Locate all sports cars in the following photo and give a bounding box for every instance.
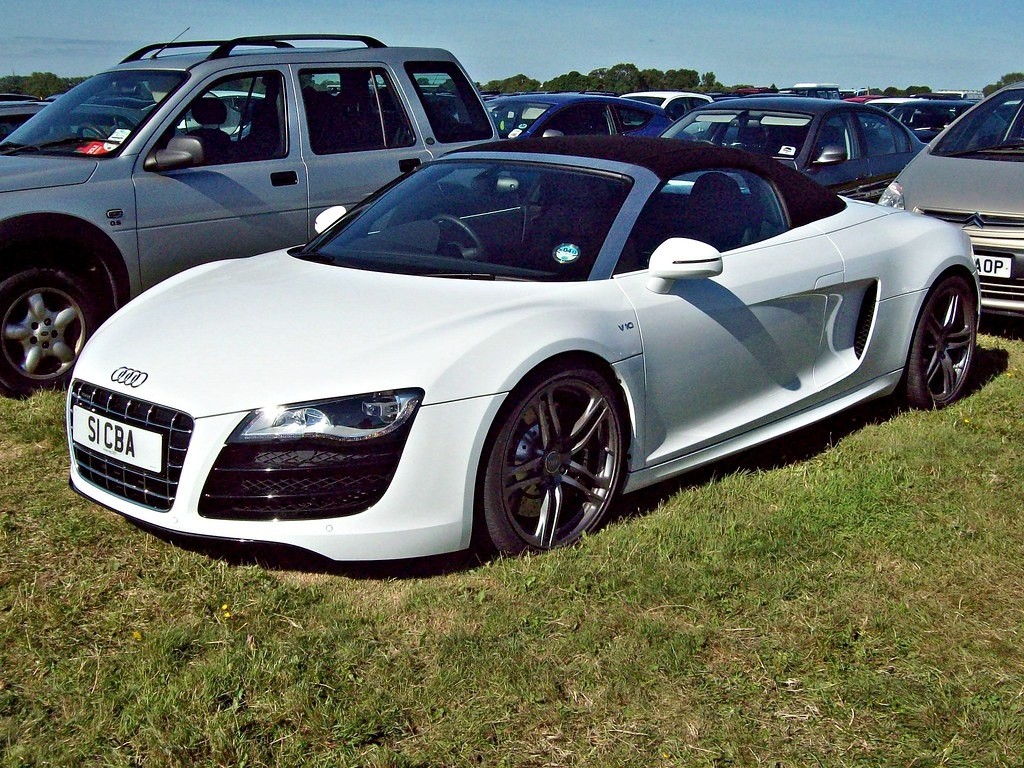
[65,134,981,564]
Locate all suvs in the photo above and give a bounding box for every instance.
[0,33,509,402]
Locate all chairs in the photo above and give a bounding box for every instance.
[672,104,685,120]
[736,127,768,153]
[185,98,234,159]
[794,126,823,160]
[225,98,280,161]
[684,171,748,251]
[480,190,535,256]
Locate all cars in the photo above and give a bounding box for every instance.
[0,74,1023,150]
[641,95,929,204]
[876,79,1023,321]
